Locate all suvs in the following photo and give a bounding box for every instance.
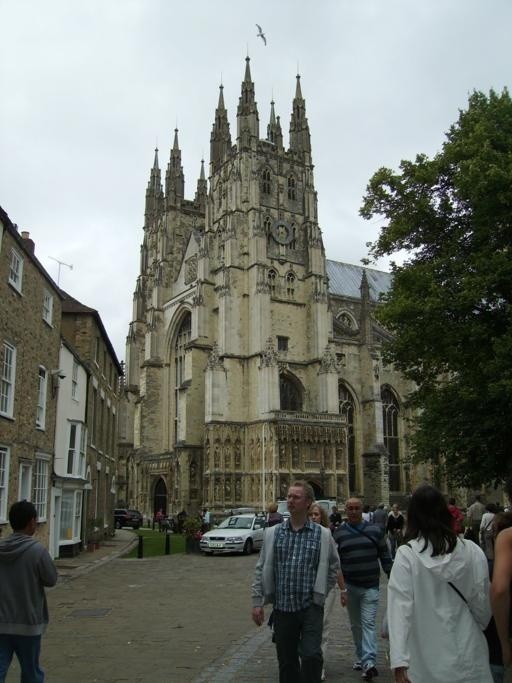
[114,509,143,530]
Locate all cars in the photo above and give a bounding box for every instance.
[199,512,268,556]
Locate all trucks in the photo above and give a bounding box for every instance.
[276,500,373,524]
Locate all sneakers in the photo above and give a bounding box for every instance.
[352,658,379,681]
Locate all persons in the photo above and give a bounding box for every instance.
[200,507,211,529]
[155,509,163,520]
[0,499,57,683]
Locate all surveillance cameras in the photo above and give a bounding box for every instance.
[57,371,66,379]
